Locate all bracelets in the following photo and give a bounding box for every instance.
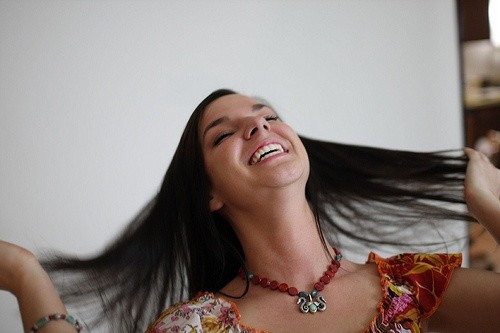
[25,312,83,333]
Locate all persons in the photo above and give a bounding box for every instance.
[1,88,499,332]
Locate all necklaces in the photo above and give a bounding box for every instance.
[247,247,343,313]
